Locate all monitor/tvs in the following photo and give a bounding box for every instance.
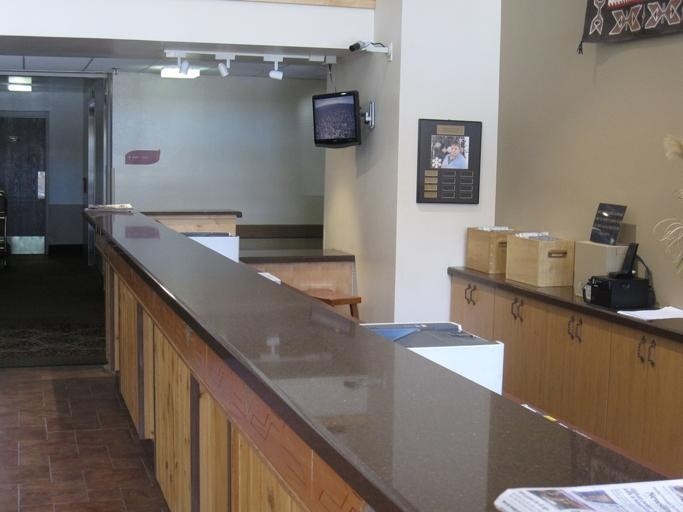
[312,91,375,148]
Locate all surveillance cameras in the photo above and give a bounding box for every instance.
[349,40,371,53]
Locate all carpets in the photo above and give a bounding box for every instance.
[0,243,108,370]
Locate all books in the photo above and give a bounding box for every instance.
[617,305,683,322]
[368,325,417,344]
[491,478,683,512]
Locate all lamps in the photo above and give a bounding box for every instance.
[175,51,285,80]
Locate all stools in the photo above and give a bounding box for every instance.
[304,287,361,320]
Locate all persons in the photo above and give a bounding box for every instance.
[438,140,467,171]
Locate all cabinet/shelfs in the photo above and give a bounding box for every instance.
[450,274,683,481]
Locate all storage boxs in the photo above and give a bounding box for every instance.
[465,227,574,288]
